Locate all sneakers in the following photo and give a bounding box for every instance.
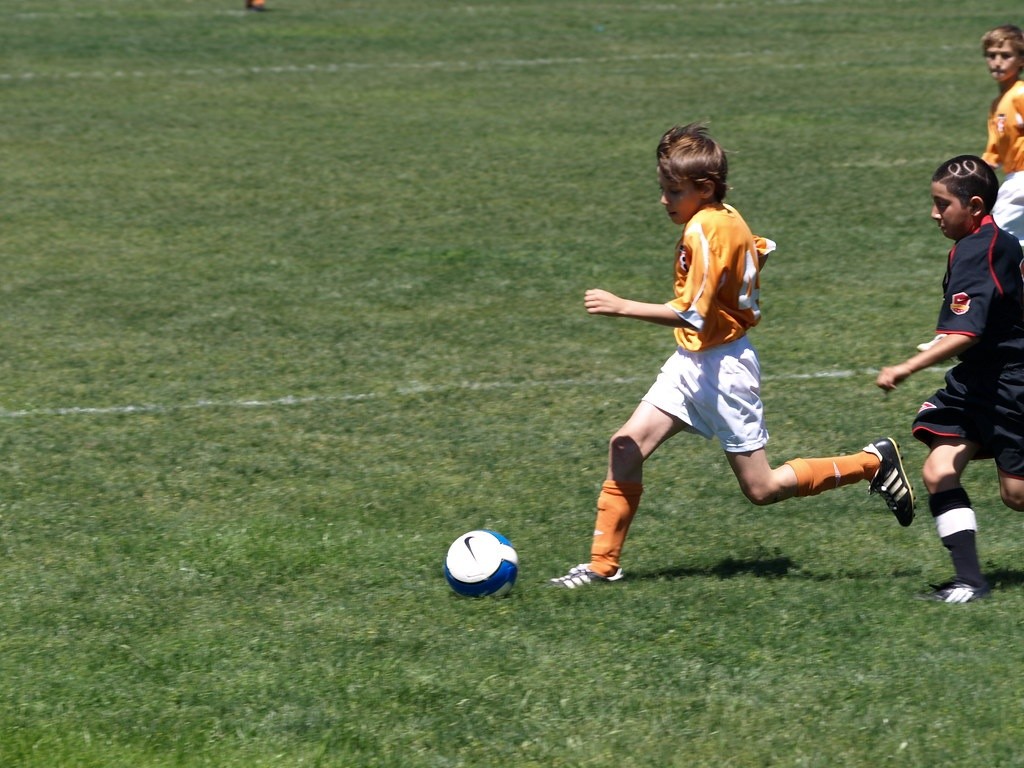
[914,576,987,603]
[550,563,623,588]
[863,437,916,527]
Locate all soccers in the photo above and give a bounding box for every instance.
[445,530,518,598]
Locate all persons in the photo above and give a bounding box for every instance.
[876,155,1024,602]
[551,124,916,591]
[914,25,1024,352]
